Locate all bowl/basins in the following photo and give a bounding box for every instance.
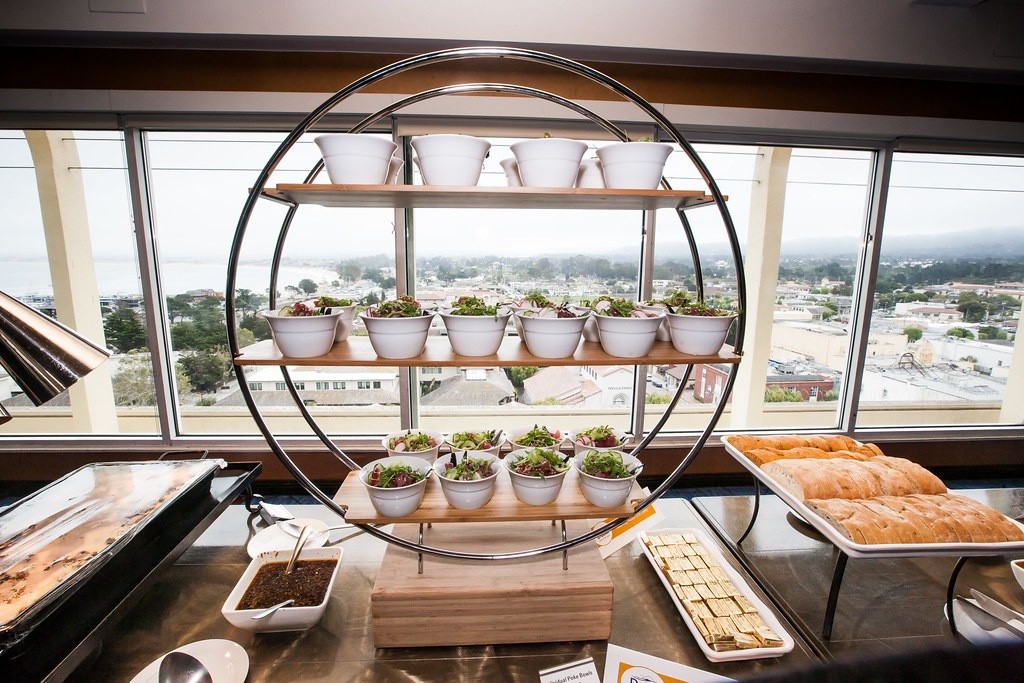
[1011,559,1024,591]
[313,133,674,191]
[358,428,644,518]
[261,298,741,359]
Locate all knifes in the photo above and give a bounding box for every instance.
[956,595,1024,640]
[970,588,1024,627]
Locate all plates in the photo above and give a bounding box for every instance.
[944,598,1024,647]
[720,433,1024,560]
[220,546,344,634]
[247,519,330,559]
[128,639,249,683]
[635,528,795,662]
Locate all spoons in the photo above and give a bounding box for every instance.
[158,652,213,683]
[276,520,356,539]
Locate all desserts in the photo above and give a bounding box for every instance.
[639,531,783,651]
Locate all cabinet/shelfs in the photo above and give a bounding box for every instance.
[226,45,746,656]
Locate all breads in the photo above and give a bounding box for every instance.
[727,433,1024,545]
